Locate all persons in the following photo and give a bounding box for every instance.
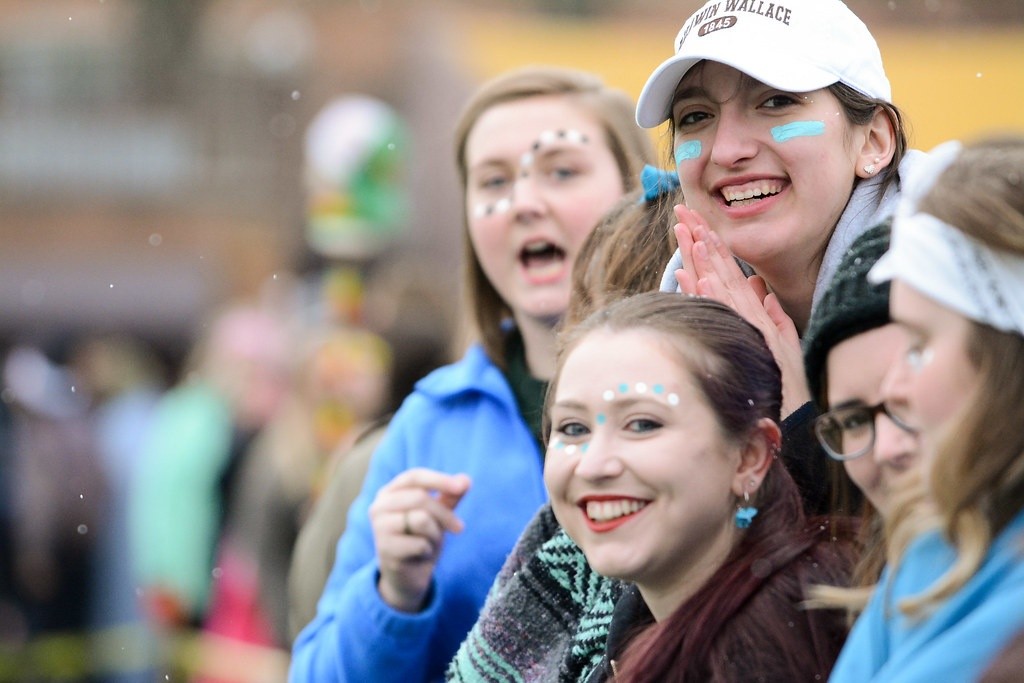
[543,292,867,682]
[451,163,760,683]
[635,1,912,519]
[0,91,452,683]
[288,70,659,682]
[804,215,926,622]
[827,146,1024,683]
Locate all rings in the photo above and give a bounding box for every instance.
[404,510,412,535]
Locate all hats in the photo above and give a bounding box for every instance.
[635,0,893,128]
[803,222,891,413]
[301,95,413,256]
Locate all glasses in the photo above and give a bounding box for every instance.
[810,399,917,462]
